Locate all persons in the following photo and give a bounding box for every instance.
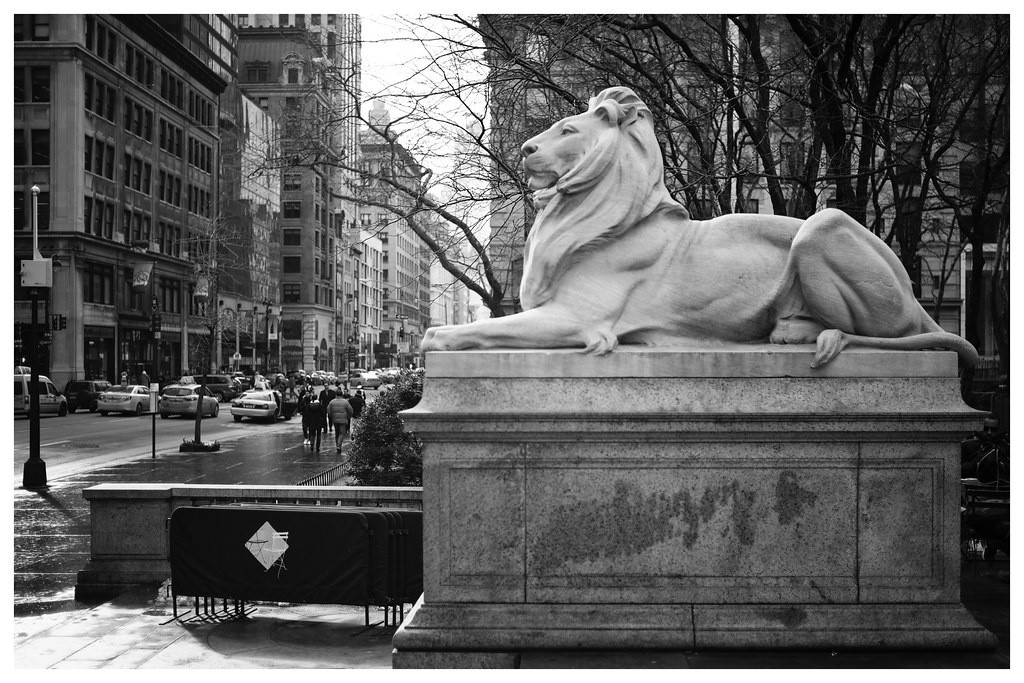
[961,415,1010,557]
[140,371,386,453]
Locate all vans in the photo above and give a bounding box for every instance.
[15,365,69,417]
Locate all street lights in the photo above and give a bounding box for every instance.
[18,184,53,488]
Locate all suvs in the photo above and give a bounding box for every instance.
[63,379,112,413]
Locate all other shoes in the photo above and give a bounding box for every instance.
[316,448,320,451]
[303,439,310,444]
[337,448,341,450]
[311,443,314,452]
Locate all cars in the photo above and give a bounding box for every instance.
[230,389,301,423]
[344,373,381,389]
[185,363,400,402]
[97,384,161,416]
[161,376,219,418]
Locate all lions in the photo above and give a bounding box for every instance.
[421,83,983,378]
